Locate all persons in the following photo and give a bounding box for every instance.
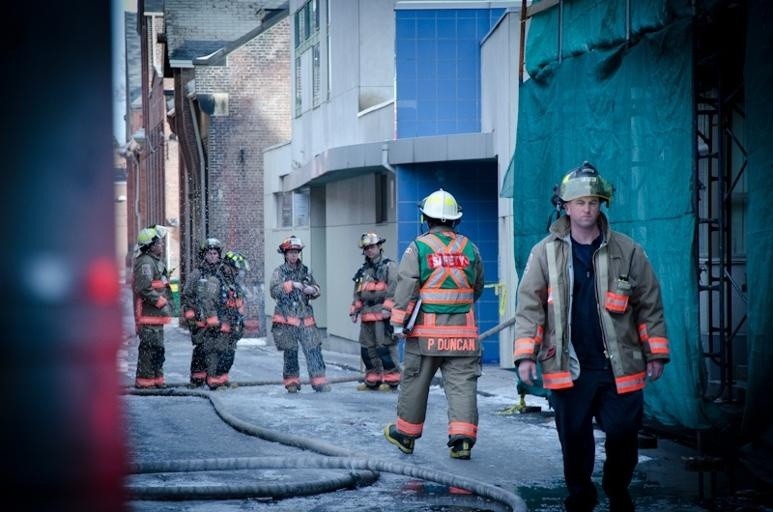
[182,237,223,386]
[205,250,251,389]
[269,235,333,392]
[385,187,485,460]
[513,161,673,511]
[131,224,176,389]
[349,233,401,391]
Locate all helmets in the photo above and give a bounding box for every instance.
[224,250,251,272]
[200,238,223,257]
[418,189,464,220]
[557,161,613,202]
[134,225,168,253]
[359,233,386,248]
[280,236,302,254]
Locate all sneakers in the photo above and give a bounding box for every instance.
[315,384,331,391]
[191,378,238,390]
[358,382,398,391]
[288,384,298,392]
[384,423,414,454]
[450,440,471,459]
[135,382,170,389]
